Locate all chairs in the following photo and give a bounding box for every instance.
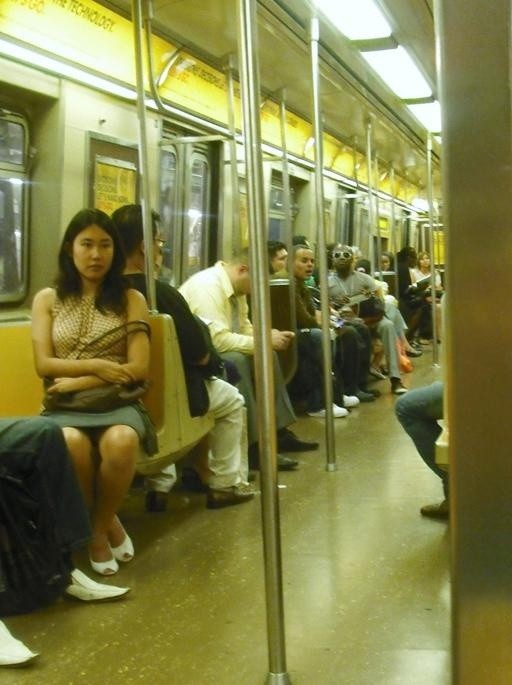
[0,256,450,494]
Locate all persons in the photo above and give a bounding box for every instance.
[109,204,253,513]
[173,248,322,476]
[2,417,133,672]
[260,239,443,419]
[27,204,155,580]
[392,376,450,517]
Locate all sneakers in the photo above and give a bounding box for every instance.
[343,395,360,407]
[307,403,348,418]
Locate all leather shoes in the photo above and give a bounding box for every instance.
[420,500,449,517]
[355,391,375,401]
[108,514,134,562]
[0,619,41,667]
[364,389,380,397]
[391,384,408,394]
[249,445,298,469]
[88,541,119,575]
[207,486,254,510]
[145,492,167,511]
[276,431,319,450]
[61,568,131,601]
[369,350,422,379]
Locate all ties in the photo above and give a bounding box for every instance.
[230,296,239,333]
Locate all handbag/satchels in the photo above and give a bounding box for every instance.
[350,297,385,326]
[42,322,151,412]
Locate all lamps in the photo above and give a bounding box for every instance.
[309,0,440,145]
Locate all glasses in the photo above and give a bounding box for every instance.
[332,252,352,261]
[154,239,167,247]
